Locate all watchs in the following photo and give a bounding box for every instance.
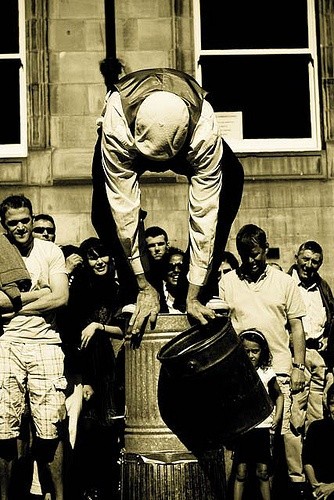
[292,364,305,370]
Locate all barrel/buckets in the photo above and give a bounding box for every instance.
[122,312,227,500]
[157,316,275,442]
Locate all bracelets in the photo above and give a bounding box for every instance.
[64,272,70,279]
[271,422,278,429]
[100,323,105,331]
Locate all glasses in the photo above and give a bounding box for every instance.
[32,227,55,234]
[5,218,30,227]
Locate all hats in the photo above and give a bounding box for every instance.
[135,91,189,160]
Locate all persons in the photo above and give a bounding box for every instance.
[0,194,334,500]
[90,67,245,334]
